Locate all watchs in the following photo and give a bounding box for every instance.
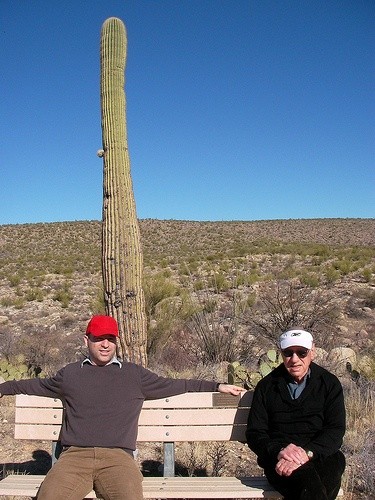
[302,446,315,462]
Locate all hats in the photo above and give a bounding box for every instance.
[86,315,118,337]
[280,330,314,349]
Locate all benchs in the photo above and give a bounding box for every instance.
[0,388,286,499]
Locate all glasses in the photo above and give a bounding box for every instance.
[281,348,309,357]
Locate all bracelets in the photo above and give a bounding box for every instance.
[216,383,220,392]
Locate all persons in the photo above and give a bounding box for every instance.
[245,326,346,500]
[0,316,247,500]
